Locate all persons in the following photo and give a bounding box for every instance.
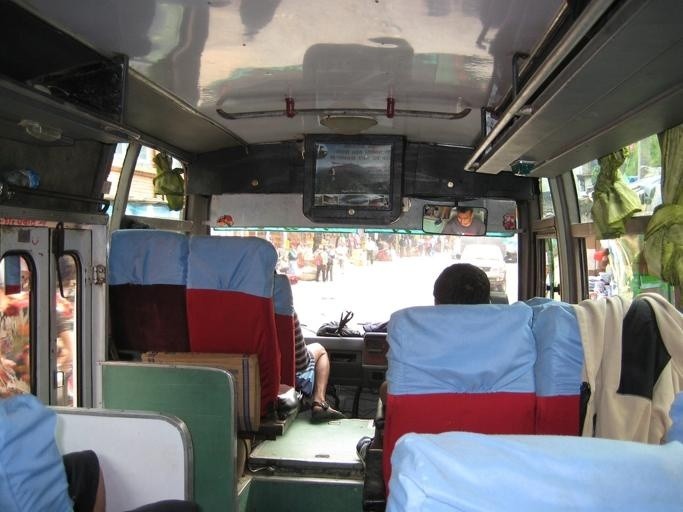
[356,263,493,481]
[0,351,204,512]
[273,269,348,420]
[264,235,465,282]
[440,207,486,235]
[595,238,637,298]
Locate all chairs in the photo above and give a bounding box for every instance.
[107,230,304,441]
[362,297,682,512]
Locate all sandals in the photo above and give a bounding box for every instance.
[312,401,345,419]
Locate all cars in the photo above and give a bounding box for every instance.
[454,236,518,307]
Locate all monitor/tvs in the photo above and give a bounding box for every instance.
[303,135,404,224]
